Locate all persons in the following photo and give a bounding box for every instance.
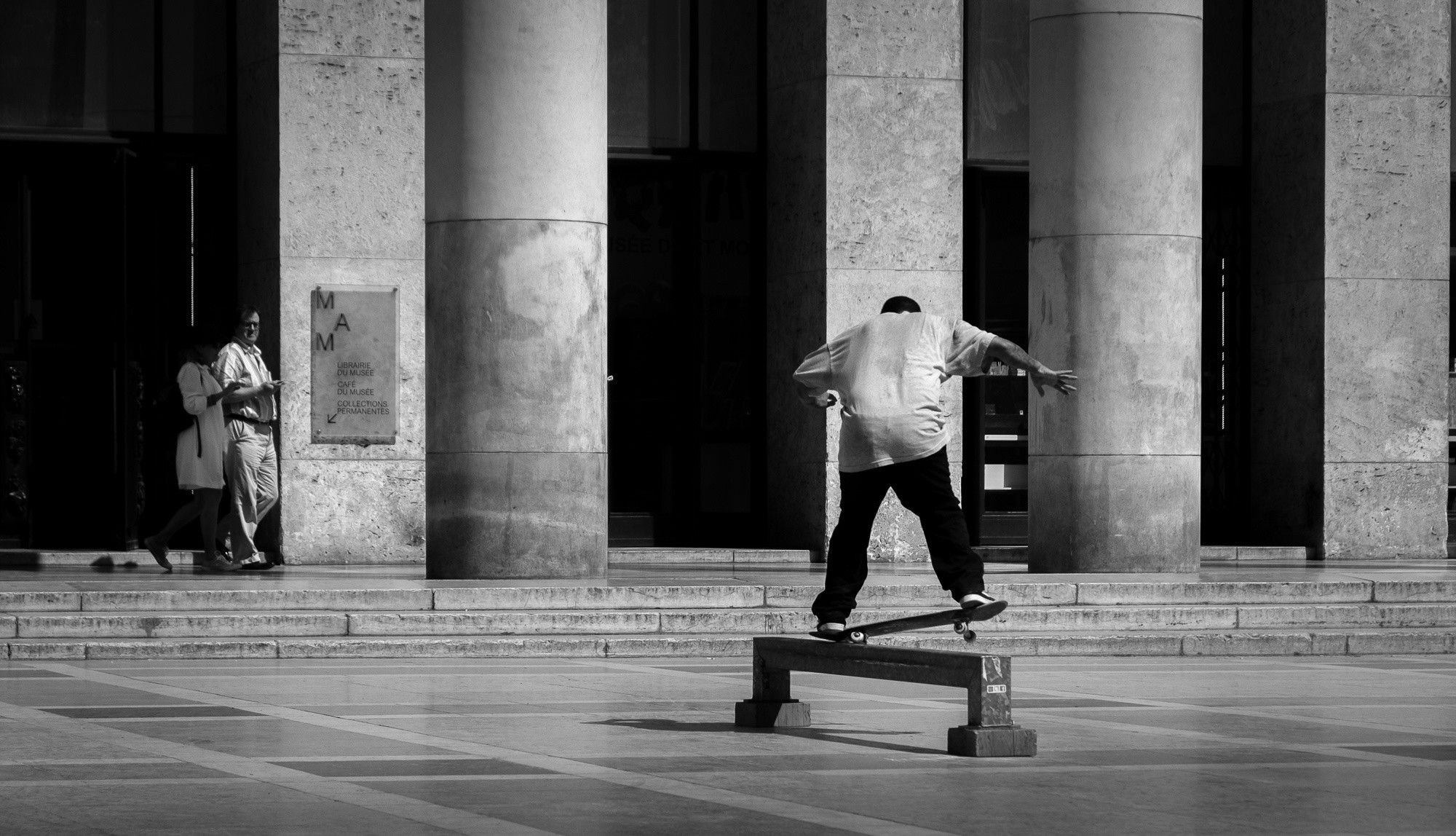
[210,304,284,570]
[792,296,1079,631]
[143,325,242,571]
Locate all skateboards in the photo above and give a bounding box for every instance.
[808,600,1009,645]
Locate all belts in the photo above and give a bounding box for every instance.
[229,412,269,425]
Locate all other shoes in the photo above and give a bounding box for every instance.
[816,619,845,632]
[242,561,272,571]
[960,591,997,609]
[216,538,231,561]
[144,537,173,569]
[206,556,242,571]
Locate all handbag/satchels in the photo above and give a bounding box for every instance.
[164,360,204,434]
